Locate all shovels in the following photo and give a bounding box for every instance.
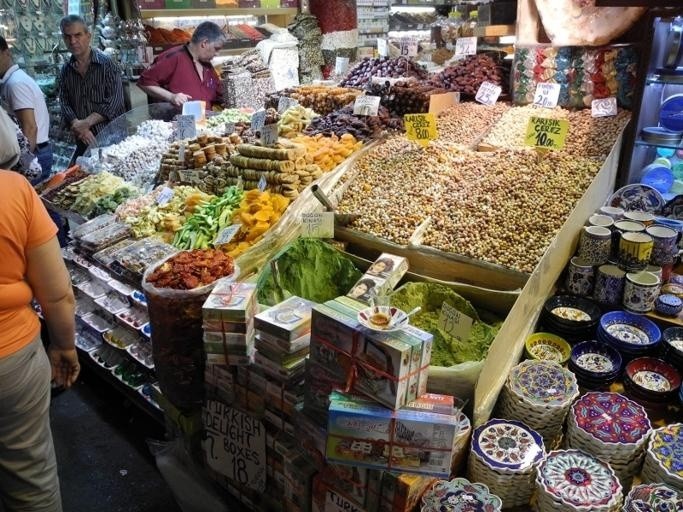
[310,185,362,227]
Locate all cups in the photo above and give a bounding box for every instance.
[563,206,682,315]
[369,286,390,322]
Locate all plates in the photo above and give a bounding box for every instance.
[417,360,683,511]
[608,184,666,215]
[357,305,408,332]
[661,194,682,249]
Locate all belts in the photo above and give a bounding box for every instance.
[34,142,48,149]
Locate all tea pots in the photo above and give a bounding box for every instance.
[95,12,150,64]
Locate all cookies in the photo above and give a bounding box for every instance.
[155,135,323,199]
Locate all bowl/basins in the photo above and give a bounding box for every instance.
[637,164,675,194]
[525,293,682,406]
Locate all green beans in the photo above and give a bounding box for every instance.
[172,182,244,252]
[331,102,631,277]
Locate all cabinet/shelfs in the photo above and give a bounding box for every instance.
[137,4,305,64]
[616,6,682,219]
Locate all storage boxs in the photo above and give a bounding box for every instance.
[192,246,472,512]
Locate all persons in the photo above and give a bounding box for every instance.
[0,35,68,248]
[0,94,42,183]
[58,15,127,166]
[136,22,226,122]
[0,169,81,512]
